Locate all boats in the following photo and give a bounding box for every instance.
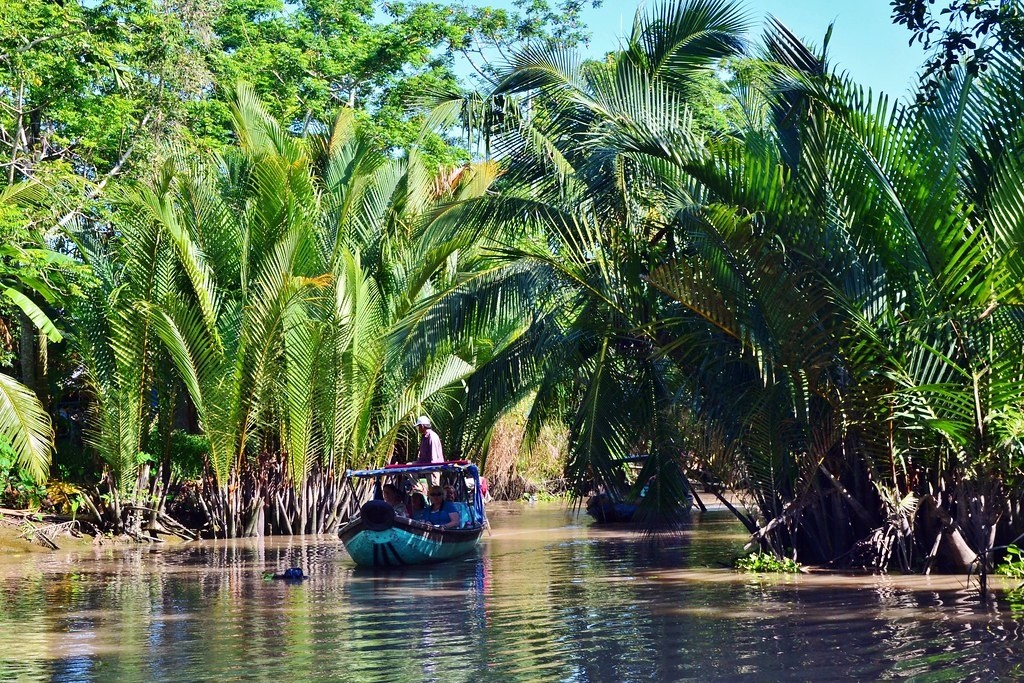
[588,454,693,528]
[337,462,491,566]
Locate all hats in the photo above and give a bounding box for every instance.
[413,416,430,426]
[401,479,415,486]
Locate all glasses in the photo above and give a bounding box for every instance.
[404,485,415,491]
[430,492,442,497]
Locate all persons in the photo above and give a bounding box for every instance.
[383,484,459,530]
[612,469,631,496]
[406,415,444,496]
